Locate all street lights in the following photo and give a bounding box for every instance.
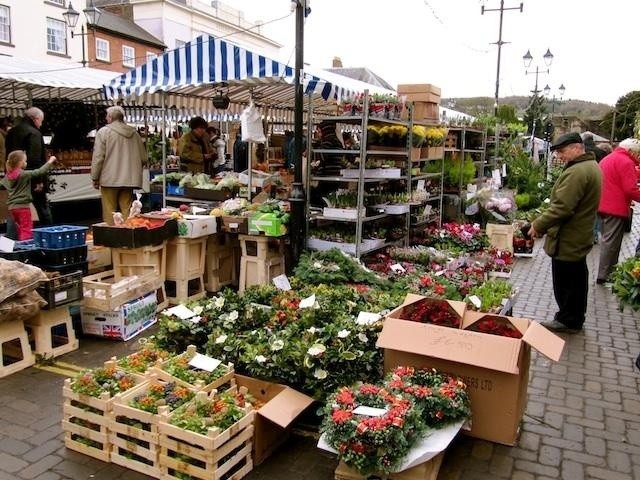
[522,48,554,137]
[62,0,102,67]
[543,84,565,145]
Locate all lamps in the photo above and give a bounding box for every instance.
[209,81,233,112]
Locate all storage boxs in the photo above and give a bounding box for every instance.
[371,290,566,451]
[1,218,293,380]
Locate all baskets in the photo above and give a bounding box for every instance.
[31,223,90,247]
[38,259,90,277]
[34,268,85,309]
[35,245,89,265]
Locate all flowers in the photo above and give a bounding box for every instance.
[323,368,471,479]
[62,345,315,479]
[288,216,520,434]
[157,278,288,381]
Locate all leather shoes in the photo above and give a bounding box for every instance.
[540,317,583,335]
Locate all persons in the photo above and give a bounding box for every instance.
[526,132,602,333]
[546,131,640,285]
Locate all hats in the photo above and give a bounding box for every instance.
[188,116,208,130]
[550,132,584,152]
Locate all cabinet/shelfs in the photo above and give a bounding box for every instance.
[0,166,294,223]
[294,84,511,263]
